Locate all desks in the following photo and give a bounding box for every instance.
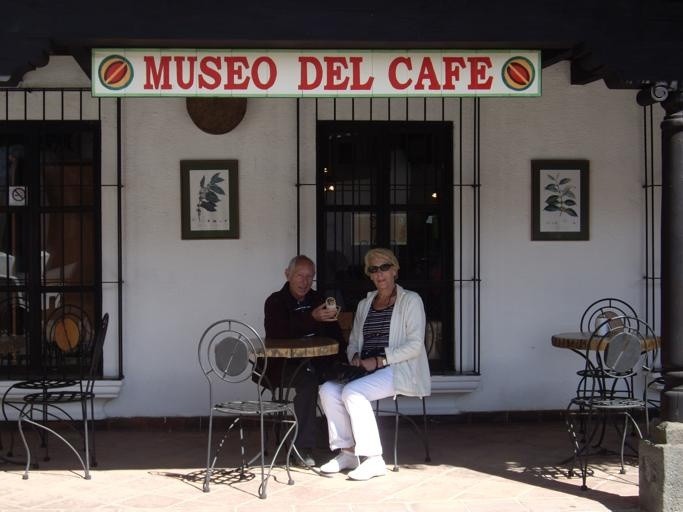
[242,336,339,465]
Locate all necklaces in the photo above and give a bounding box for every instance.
[373,288,396,337]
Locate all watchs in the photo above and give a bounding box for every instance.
[381,355,387,367]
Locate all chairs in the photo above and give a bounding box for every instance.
[198,319,299,499]
[551,298,661,490]
[367,317,435,472]
[0,296,109,480]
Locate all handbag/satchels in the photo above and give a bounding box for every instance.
[319,352,379,383]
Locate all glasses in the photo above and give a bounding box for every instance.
[367,264,394,274]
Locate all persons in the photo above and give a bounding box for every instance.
[318,248,431,480]
[251,255,347,467]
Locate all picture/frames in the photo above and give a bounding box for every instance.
[180,161,239,240]
[531,159,590,241]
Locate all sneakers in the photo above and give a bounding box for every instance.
[320,453,361,473]
[348,459,387,480]
[291,447,315,467]
